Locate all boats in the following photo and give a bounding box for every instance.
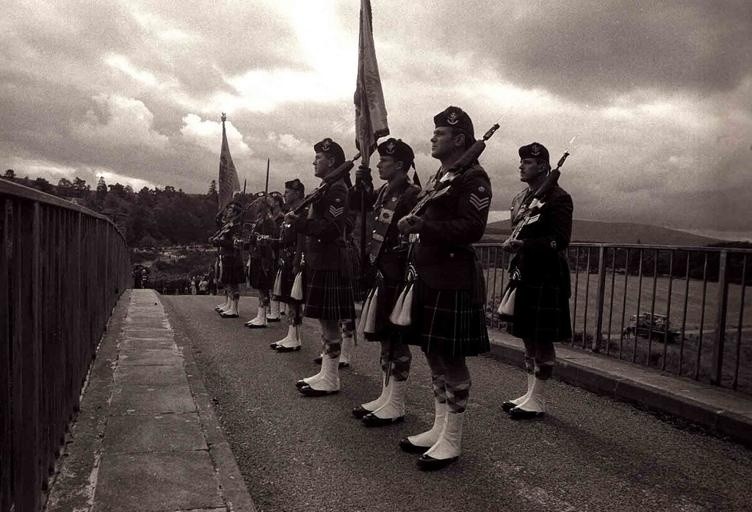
[630,312,680,341]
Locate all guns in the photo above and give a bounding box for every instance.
[406,123,501,224]
[509,152,571,240]
[292,152,363,213]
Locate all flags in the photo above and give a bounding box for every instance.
[352,2,391,167]
[214,114,243,211]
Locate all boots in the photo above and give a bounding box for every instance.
[244,299,290,328]
[295,336,355,396]
[214,296,239,317]
[400,401,465,468]
[503,373,547,418]
[352,373,409,427]
[270,324,302,351]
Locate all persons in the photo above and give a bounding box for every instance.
[214,176,373,369]
[617,314,673,342]
[498,142,572,421]
[396,104,494,472]
[351,138,422,426]
[126,242,215,307]
[283,139,355,396]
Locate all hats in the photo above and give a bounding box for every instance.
[518,143,549,162]
[313,140,345,166]
[378,138,414,165]
[285,179,304,192]
[434,106,474,136]
[227,191,282,212]
